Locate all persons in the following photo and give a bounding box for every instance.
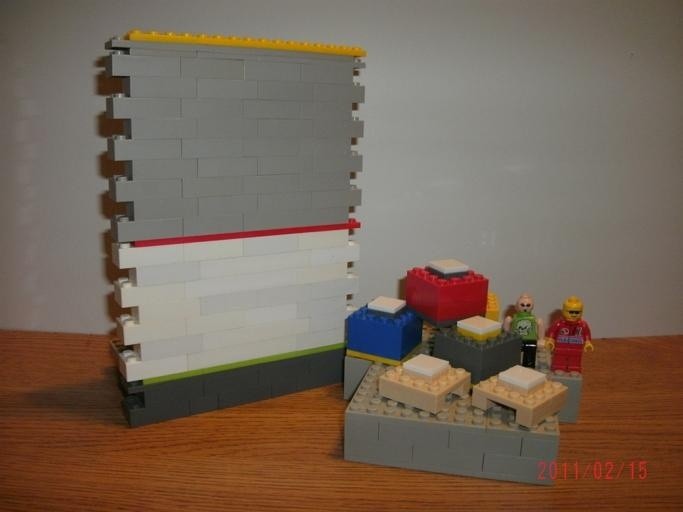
[545,296,594,377]
[503,291,545,369]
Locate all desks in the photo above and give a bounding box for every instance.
[0,330,683,512]
